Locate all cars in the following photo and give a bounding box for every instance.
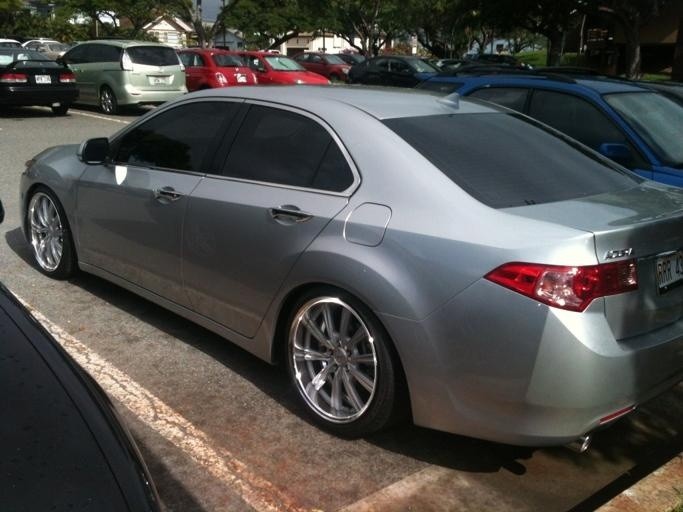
[22,40,71,60]
[57,40,189,114]
[335,53,367,66]
[1,48,80,115]
[347,55,448,91]
[177,47,258,91]
[1,38,22,47]
[16,80,683,445]
[234,51,332,86]
[438,54,533,75]
[409,71,683,192]
[292,52,352,80]
